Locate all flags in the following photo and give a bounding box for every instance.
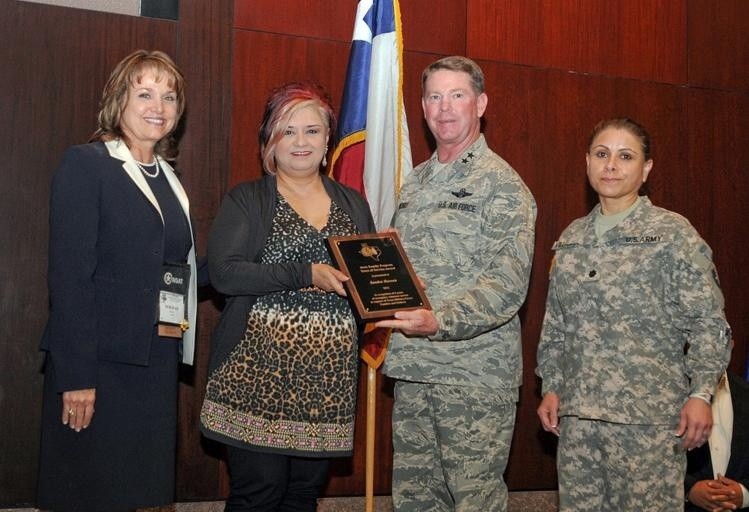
[324,0,415,369]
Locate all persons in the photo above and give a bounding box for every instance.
[198,81,426,512]
[374,54,538,512]
[682,338,749,512]
[532,118,733,512]
[34,49,212,512]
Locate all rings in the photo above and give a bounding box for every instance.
[68,409,76,416]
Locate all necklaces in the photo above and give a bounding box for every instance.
[135,154,160,178]
